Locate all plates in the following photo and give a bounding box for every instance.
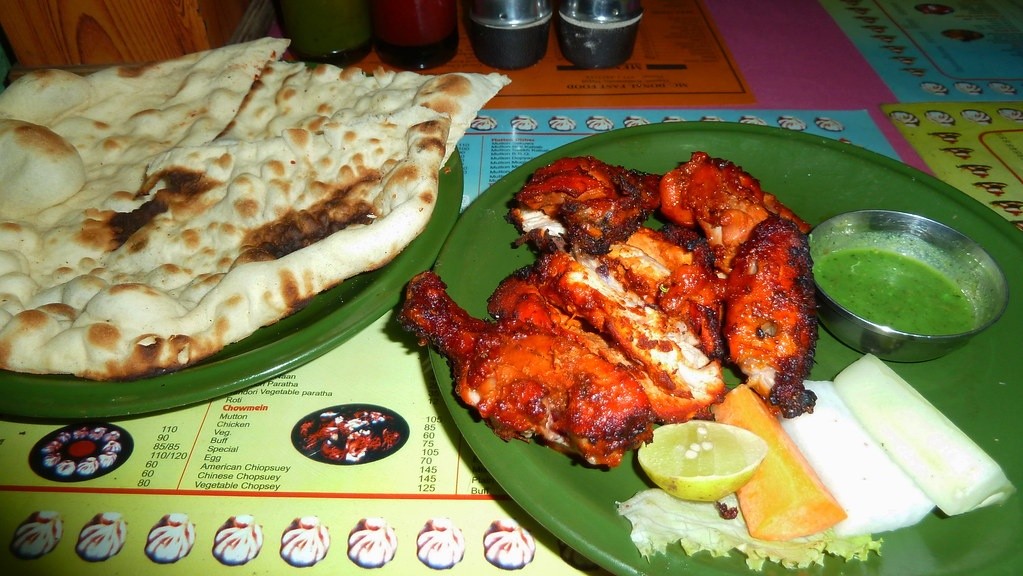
[26,421,136,485]
[0,147,463,420]
[289,404,411,466]
[427,122,1023,576]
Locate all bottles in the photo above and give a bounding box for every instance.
[462,0,553,69]
[558,0,643,69]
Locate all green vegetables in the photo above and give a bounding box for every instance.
[615,487,883,572]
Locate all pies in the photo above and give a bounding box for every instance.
[0,36,513,388]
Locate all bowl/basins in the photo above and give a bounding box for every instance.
[807,210,1009,363]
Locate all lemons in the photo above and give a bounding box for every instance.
[637,420,767,503]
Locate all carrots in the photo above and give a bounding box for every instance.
[714,383,849,541]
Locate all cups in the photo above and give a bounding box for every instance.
[270,0,374,68]
[370,0,459,70]
